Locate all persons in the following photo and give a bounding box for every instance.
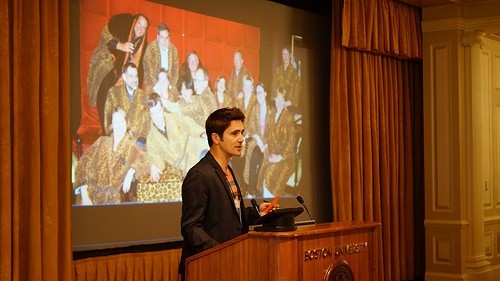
[72,10,304,205]
[177,107,281,281]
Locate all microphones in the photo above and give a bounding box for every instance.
[297,196,312,220]
[251,199,261,218]
